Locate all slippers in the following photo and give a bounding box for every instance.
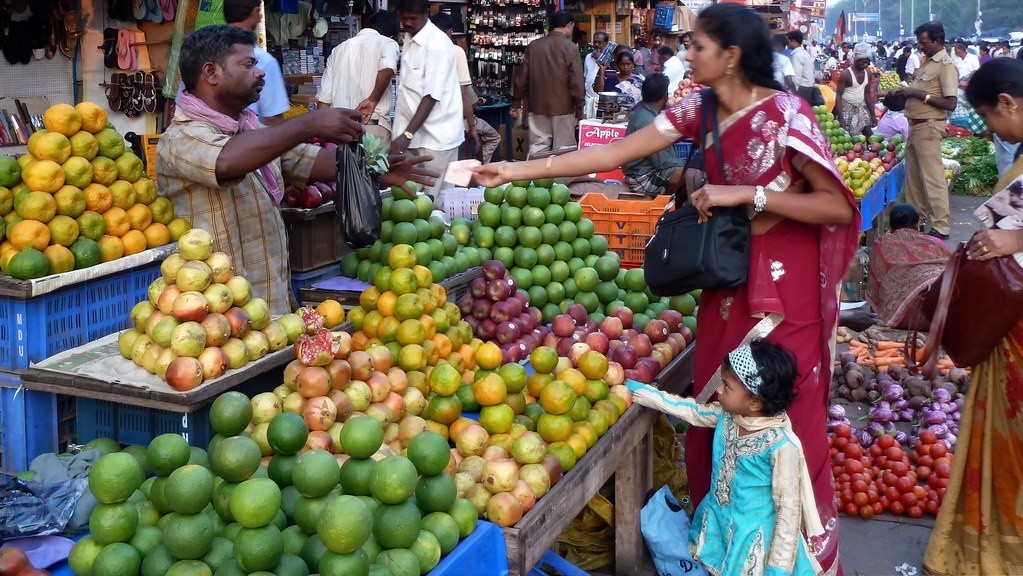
[45,0,176,118]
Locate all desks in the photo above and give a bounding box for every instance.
[851,158,905,247]
[473,99,513,162]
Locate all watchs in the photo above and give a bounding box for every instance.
[753,185,766,212]
[403,131,413,140]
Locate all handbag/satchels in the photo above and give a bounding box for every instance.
[337,141,382,247]
[644,90,749,296]
[904,231,1022,381]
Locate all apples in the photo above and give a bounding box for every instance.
[454,260,693,406]
[666,72,708,106]
[279,136,344,209]
[835,142,899,172]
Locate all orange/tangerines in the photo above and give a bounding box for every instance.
[321,243,629,473]
[16,392,478,576]
[833,158,875,198]
[811,104,905,161]
[869,68,904,91]
[0,101,193,281]
[340,178,704,337]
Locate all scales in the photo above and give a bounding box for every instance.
[595,91,633,123]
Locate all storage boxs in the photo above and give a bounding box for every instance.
[576,193,675,270]
[280,190,392,272]
[674,143,693,161]
[440,183,511,225]
[529,550,592,576]
[498,122,529,161]
[298,75,322,98]
[0,260,216,485]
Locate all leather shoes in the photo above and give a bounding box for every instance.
[920,226,925,232]
[927,229,949,240]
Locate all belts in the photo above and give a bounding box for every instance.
[908,118,947,126]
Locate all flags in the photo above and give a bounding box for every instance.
[836,8,846,44]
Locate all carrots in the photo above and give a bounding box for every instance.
[848,340,972,375]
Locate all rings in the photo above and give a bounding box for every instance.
[978,241,987,253]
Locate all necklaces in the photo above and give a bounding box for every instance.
[750,84,758,104]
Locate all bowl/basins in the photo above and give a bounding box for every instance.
[598,92,626,103]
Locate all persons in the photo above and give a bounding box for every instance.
[865,204,954,334]
[509,10,586,161]
[622,332,825,576]
[771,30,924,107]
[677,32,694,78]
[584,31,686,118]
[890,21,959,241]
[470,0,862,576]
[155,24,440,399]
[946,38,1023,126]
[922,55,1023,576]
[620,72,686,197]
[429,12,501,165]
[836,43,877,148]
[175,0,465,209]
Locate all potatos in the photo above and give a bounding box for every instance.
[832,352,972,400]
[836,326,927,347]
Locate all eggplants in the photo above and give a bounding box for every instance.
[827,384,962,451]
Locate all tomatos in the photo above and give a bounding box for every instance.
[823,423,954,519]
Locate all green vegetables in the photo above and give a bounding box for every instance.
[940,134,998,195]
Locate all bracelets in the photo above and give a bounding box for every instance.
[306,27,312,30]
[923,94,930,104]
[469,125,475,127]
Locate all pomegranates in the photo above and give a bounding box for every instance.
[118,228,324,391]
[251,327,551,527]
